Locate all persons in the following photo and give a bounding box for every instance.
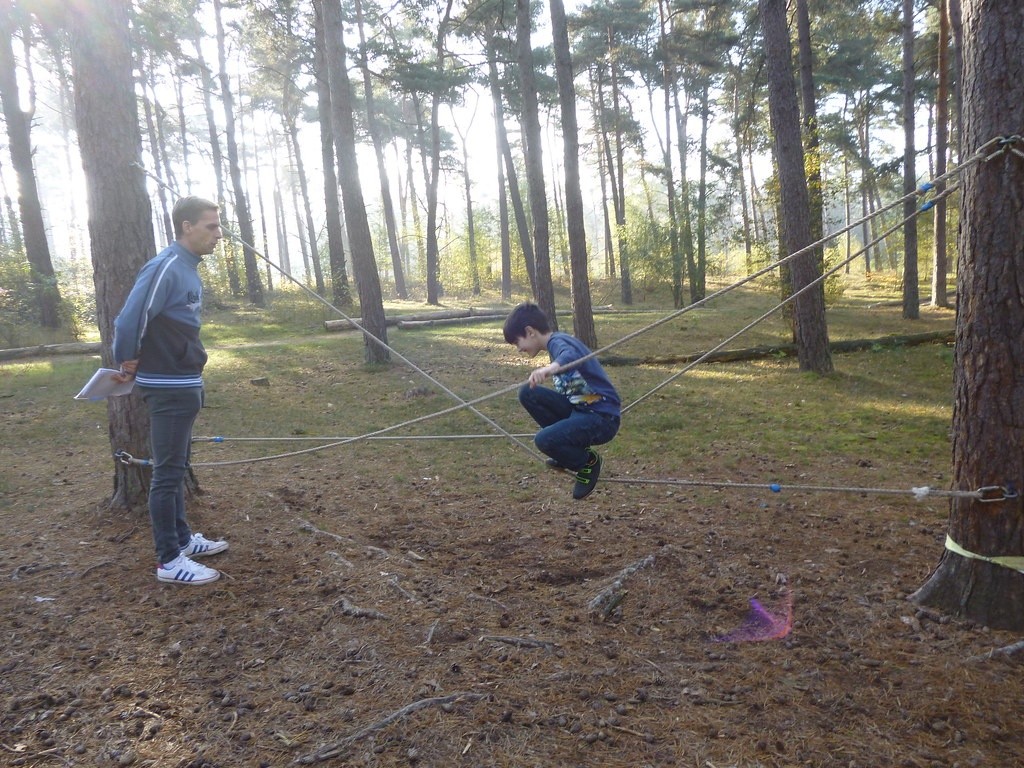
[111,196,230,587]
[502,300,623,502]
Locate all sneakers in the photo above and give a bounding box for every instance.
[546,456,578,473]
[571,448,604,500]
[180,532,230,559]
[156,556,220,586]
